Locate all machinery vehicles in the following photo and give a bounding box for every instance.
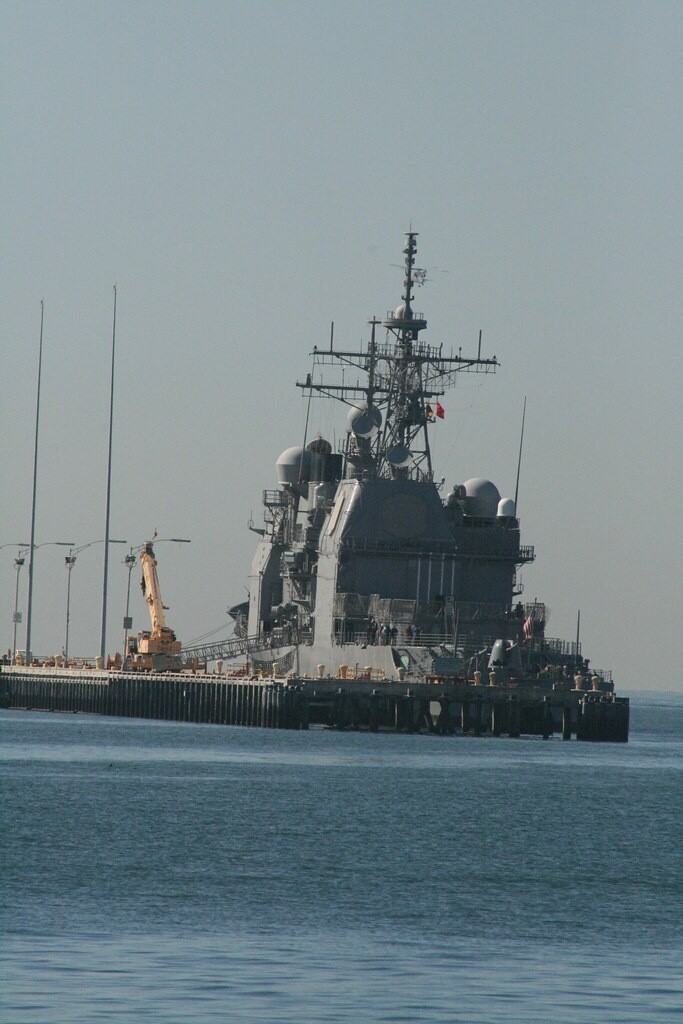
[123,539,186,673]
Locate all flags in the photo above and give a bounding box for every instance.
[436,400,444,419]
[425,402,434,418]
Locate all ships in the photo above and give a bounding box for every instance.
[203,218,616,734]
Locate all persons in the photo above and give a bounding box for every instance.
[365,615,426,647]
[515,601,525,617]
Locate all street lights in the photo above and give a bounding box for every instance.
[62,539,128,668]
[11,542,75,668]
[122,538,192,668]
